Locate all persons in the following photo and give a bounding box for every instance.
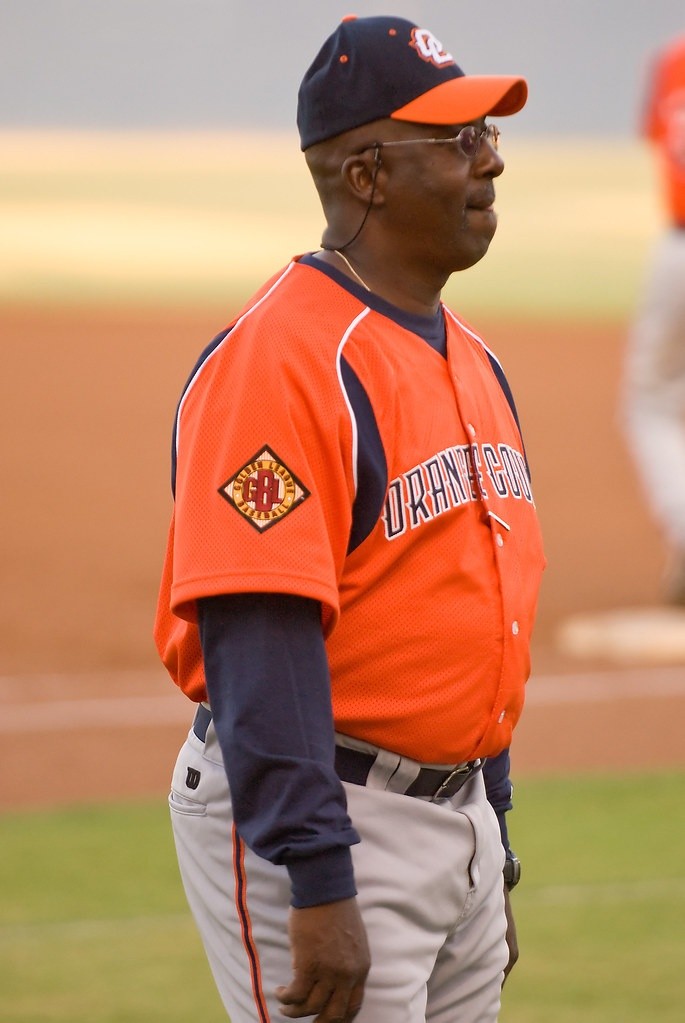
[617,35,685,607]
[151,14,545,1023]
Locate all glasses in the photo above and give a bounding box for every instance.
[356,123,500,159]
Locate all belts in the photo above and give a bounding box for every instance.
[192,702,487,802]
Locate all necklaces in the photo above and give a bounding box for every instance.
[321,244,372,294]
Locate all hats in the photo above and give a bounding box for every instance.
[297,15,528,152]
